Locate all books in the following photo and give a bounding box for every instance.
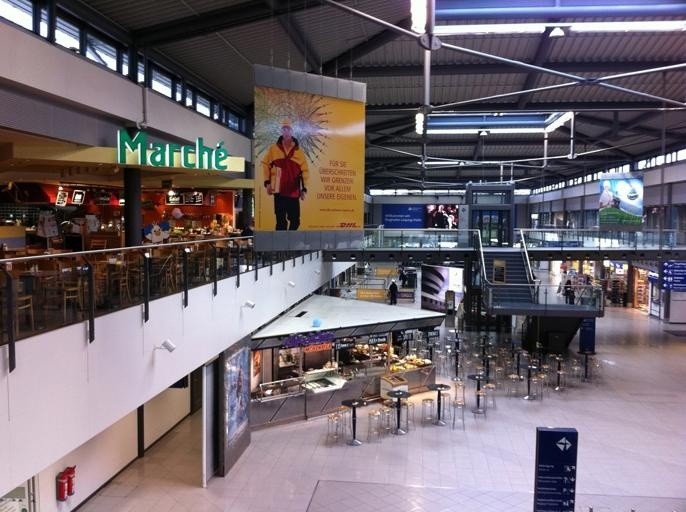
[270,167,282,194]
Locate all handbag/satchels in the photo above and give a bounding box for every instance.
[269,166,282,193]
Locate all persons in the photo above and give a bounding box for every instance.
[563,280,572,304]
[432,205,458,228]
[262,119,308,231]
[399,272,408,288]
[600,181,614,208]
[456,299,466,333]
[388,279,398,305]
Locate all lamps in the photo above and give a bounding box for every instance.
[409,0,686,136]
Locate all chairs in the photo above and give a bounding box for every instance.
[0,226,252,338]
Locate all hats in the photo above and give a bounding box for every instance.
[280,120,294,130]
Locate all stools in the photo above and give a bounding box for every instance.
[326,334,601,446]
